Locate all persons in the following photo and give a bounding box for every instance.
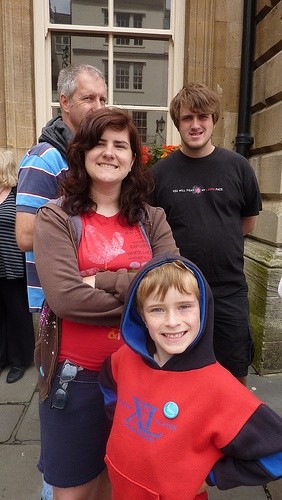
[15,64,107,500]
[149,82,262,387]
[33,107,180,500]
[97,252,282,500]
[0,148,34,384]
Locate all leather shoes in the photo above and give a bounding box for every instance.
[7,363,28,382]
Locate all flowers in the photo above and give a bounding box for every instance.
[139,144,179,167]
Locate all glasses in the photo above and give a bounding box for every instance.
[50,358,84,410]
